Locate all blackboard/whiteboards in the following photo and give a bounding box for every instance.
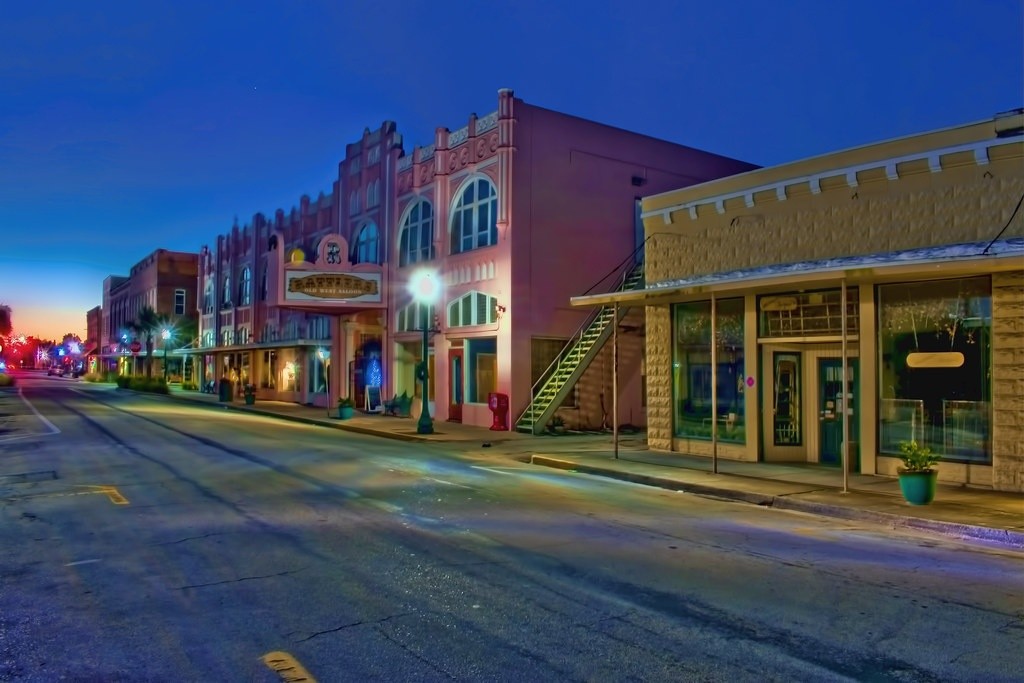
[365,385,382,412]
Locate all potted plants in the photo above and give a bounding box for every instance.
[897,440,941,505]
[338,396,354,419]
[244,383,256,405]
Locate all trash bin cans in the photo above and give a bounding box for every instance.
[219,382,234,402]
